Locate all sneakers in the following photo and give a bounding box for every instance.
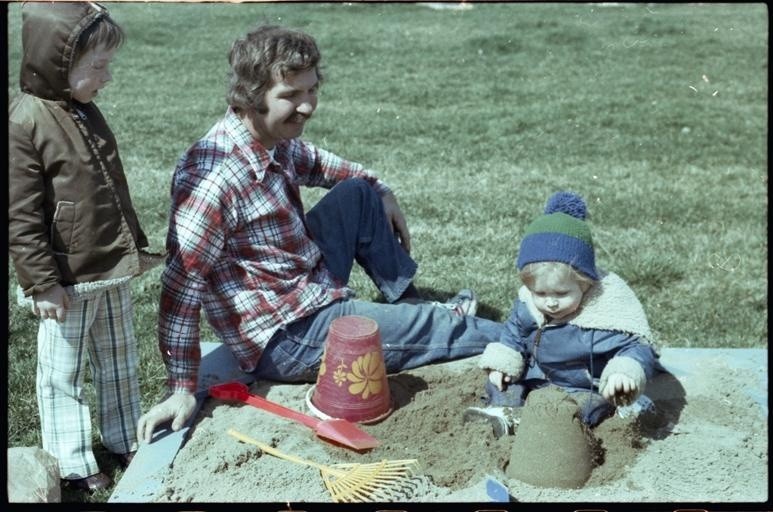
[462,401,513,438]
[439,288,479,317]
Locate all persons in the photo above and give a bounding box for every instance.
[7,1,143,493]
[462,192,670,441]
[136,27,505,446]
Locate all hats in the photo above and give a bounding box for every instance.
[515,191,601,280]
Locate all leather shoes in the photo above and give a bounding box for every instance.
[63,471,113,491]
[122,452,140,461]
